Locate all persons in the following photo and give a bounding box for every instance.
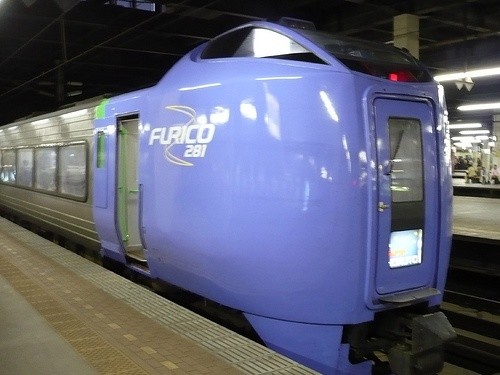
[19,160,32,187]
[489,164,500,183]
[453,155,485,171]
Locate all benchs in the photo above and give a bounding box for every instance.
[468,175,500,184]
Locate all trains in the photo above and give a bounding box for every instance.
[1,18,455,375]
